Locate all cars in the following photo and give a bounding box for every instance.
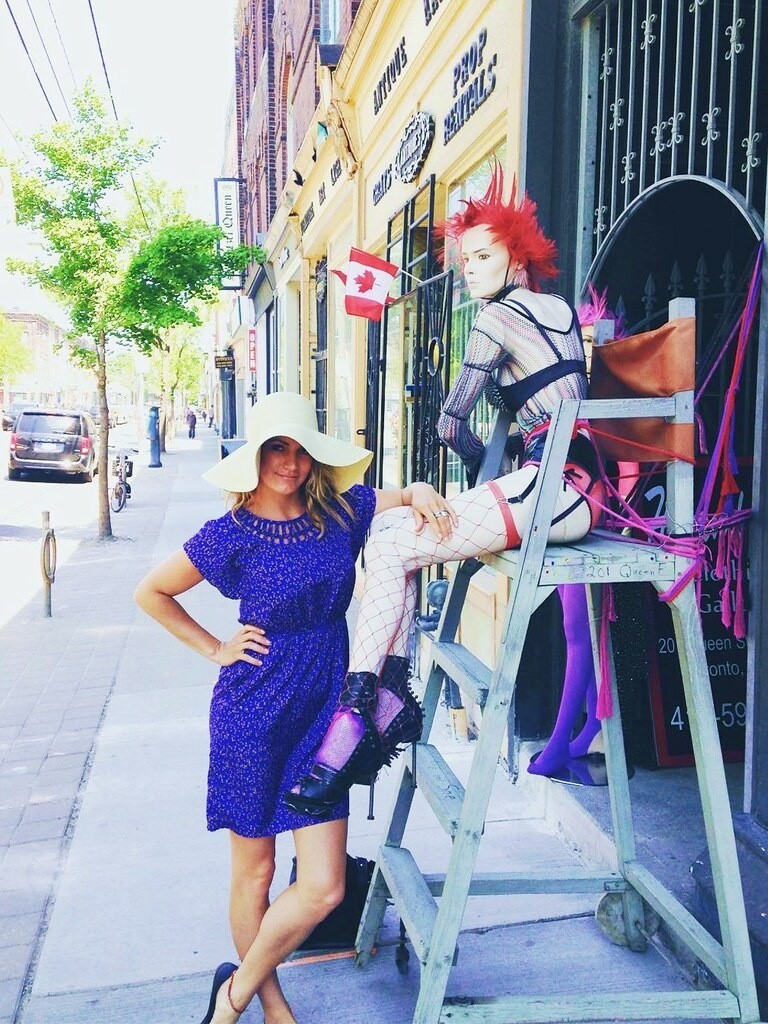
[8,408,99,484]
[1,400,136,432]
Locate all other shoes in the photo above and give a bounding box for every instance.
[201,962,239,1024]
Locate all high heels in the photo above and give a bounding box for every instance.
[279,673,386,822]
[375,655,424,789]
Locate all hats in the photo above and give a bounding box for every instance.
[200,391,375,496]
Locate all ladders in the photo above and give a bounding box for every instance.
[355,297,761,1024]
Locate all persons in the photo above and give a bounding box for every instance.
[282,150,604,818]
[528,283,639,774]
[138,392,460,1024]
[185,403,215,439]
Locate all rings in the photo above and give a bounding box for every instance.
[435,510,449,517]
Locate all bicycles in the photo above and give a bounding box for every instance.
[106,444,140,514]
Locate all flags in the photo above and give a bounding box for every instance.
[331,269,396,304]
[342,247,398,322]
